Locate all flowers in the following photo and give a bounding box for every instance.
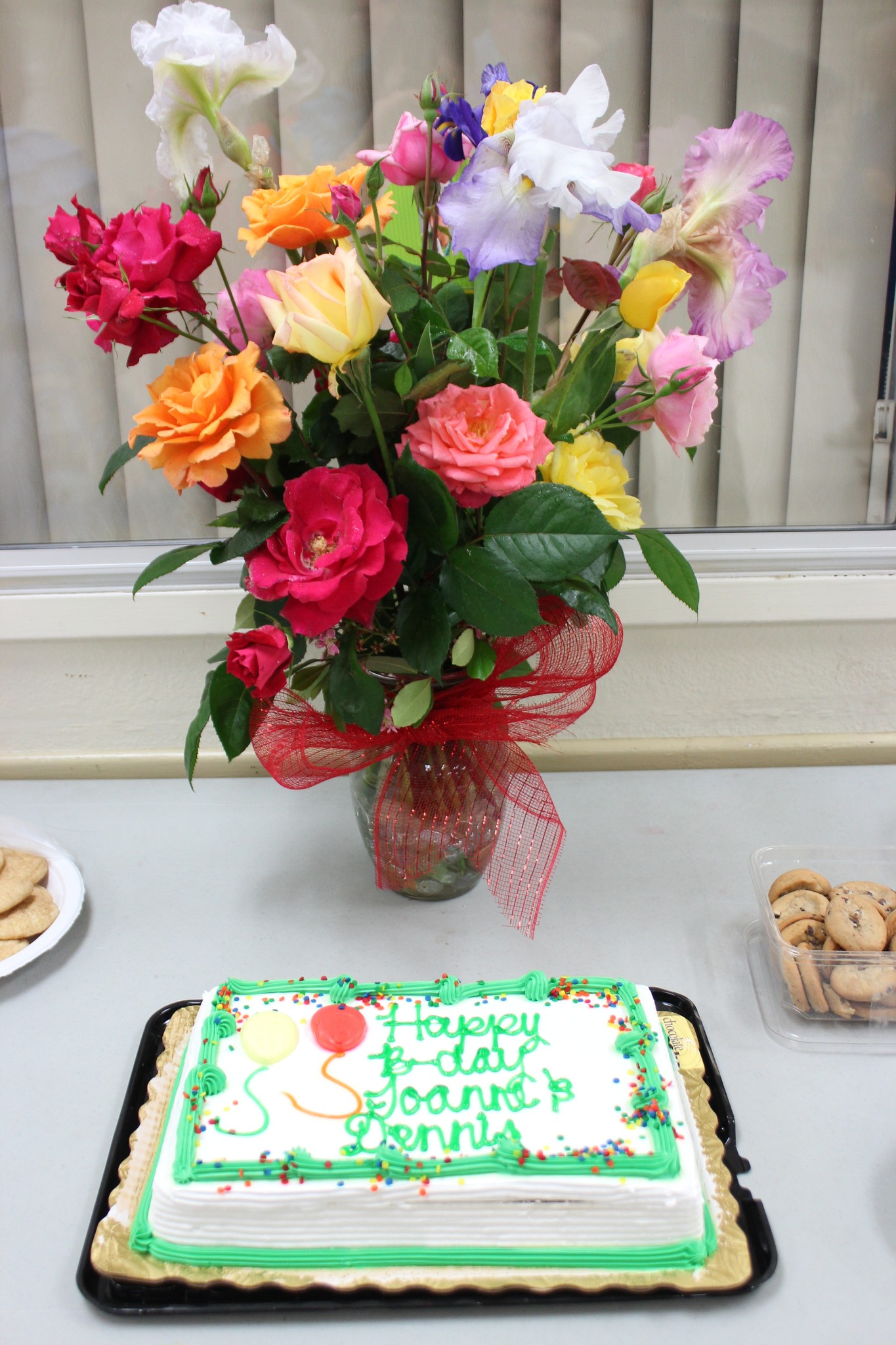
[42,0,799,801]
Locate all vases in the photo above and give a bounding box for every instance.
[331,696,525,903]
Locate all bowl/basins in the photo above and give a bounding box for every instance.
[751,842,894,1025]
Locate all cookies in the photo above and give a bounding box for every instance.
[764,860,896,1022]
[1,844,64,966]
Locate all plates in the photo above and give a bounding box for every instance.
[1,819,86,984]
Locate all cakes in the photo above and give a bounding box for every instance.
[113,964,723,1271]
